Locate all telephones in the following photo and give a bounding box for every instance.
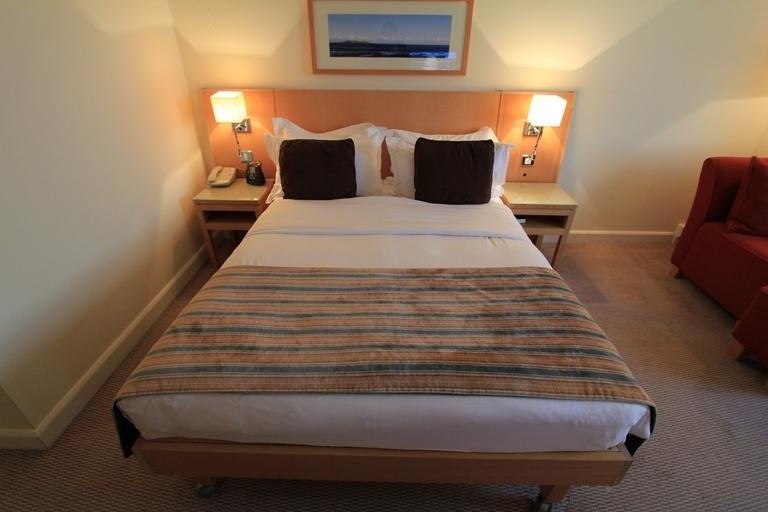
[206,165,237,187]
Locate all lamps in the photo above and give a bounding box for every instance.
[212,91,253,164]
[522,94,567,166]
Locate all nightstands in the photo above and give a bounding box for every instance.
[501,183,578,273]
[193,179,274,271]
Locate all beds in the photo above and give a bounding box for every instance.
[113,91,657,512]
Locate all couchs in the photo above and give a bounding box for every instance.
[671,156,767,363]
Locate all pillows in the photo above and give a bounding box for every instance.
[415,138,494,205]
[261,129,392,205]
[279,139,356,200]
[392,127,499,145]
[271,117,373,141]
[385,136,514,199]
[726,156,767,234]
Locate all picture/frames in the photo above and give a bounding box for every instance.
[307,0,474,76]
